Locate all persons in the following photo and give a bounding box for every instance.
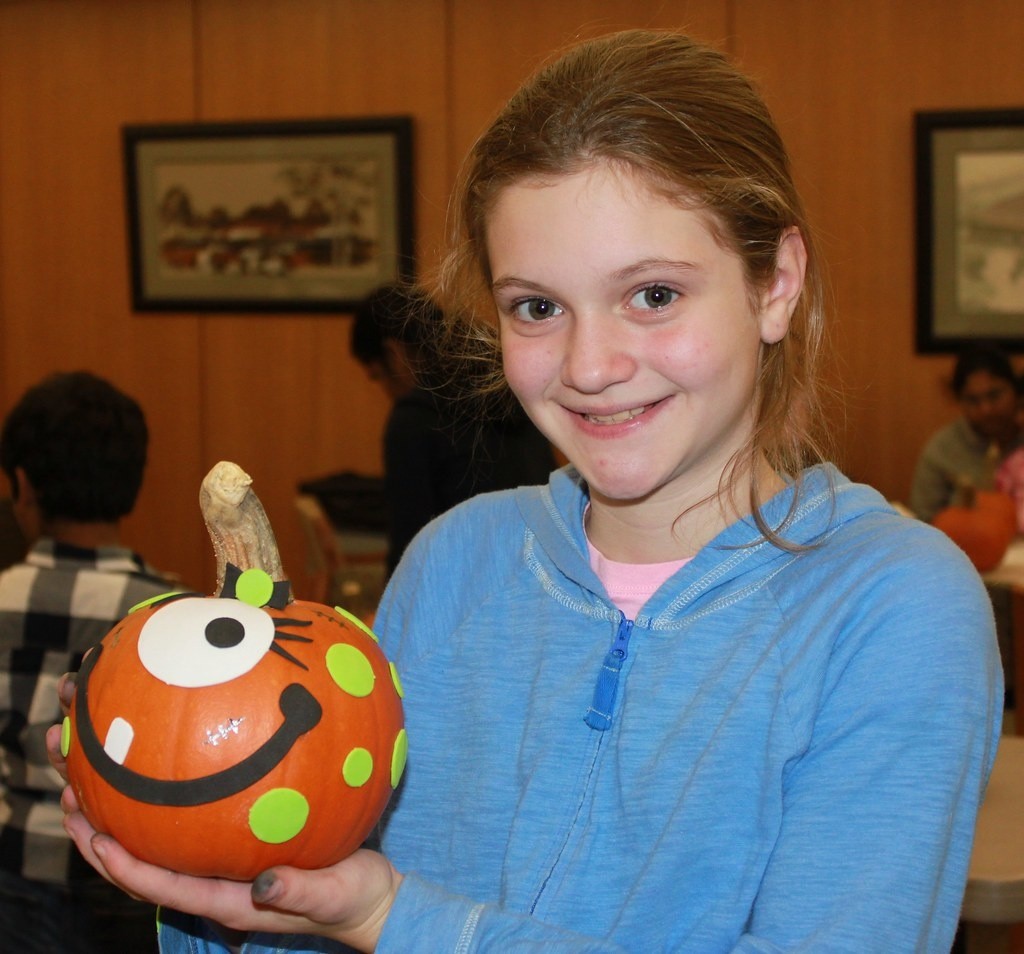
[909,345,1024,524]
[283,282,558,587]
[0,371,204,954]
[42,29,1005,954]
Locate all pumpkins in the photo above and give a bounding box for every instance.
[64,461,407,884]
[934,444,1018,573]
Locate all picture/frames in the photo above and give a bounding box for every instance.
[123,114,420,316]
[913,109,1023,356]
